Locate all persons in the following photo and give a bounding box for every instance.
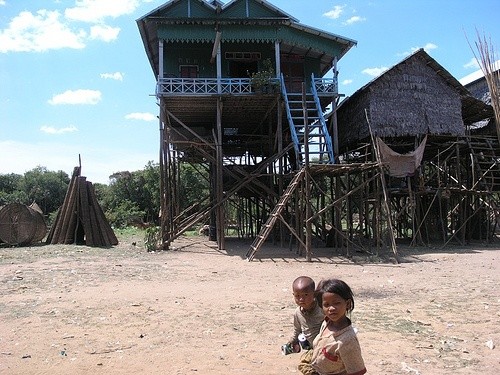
[280,276,327,375]
[310,279,367,375]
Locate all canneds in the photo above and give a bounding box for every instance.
[281,343,301,355]
[298,334,311,350]
